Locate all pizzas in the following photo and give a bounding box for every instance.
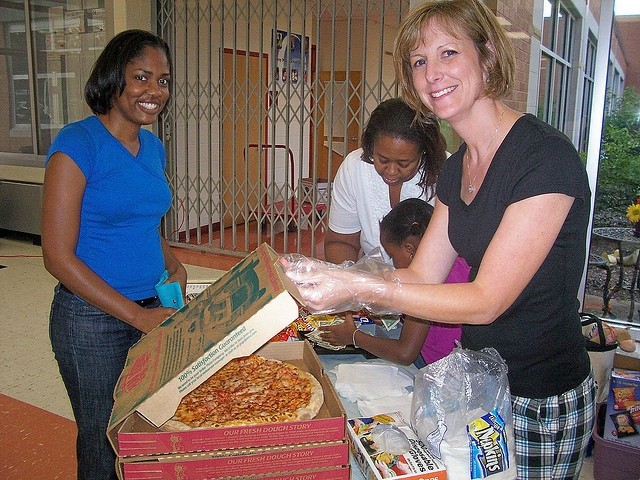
[158,353,325,434]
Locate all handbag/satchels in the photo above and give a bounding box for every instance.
[579,312,618,458]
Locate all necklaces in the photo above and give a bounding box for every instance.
[466,102,511,194]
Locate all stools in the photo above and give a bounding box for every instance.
[588,253,612,319]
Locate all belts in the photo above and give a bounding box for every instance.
[59,282,157,307]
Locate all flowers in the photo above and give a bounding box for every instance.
[625,198,640,223]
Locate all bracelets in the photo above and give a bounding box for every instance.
[352,329,361,350]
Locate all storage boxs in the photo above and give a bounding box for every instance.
[298,178,333,204]
[590,400,640,480]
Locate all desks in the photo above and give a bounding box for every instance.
[593,226,640,322]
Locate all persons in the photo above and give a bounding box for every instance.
[318,200,472,368]
[286,0,598,480]
[324,98,455,265]
[41,29,188,480]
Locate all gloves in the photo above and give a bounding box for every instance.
[307,247,397,281]
[275,252,402,317]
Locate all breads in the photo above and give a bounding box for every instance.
[615,327,633,340]
[619,338,637,352]
